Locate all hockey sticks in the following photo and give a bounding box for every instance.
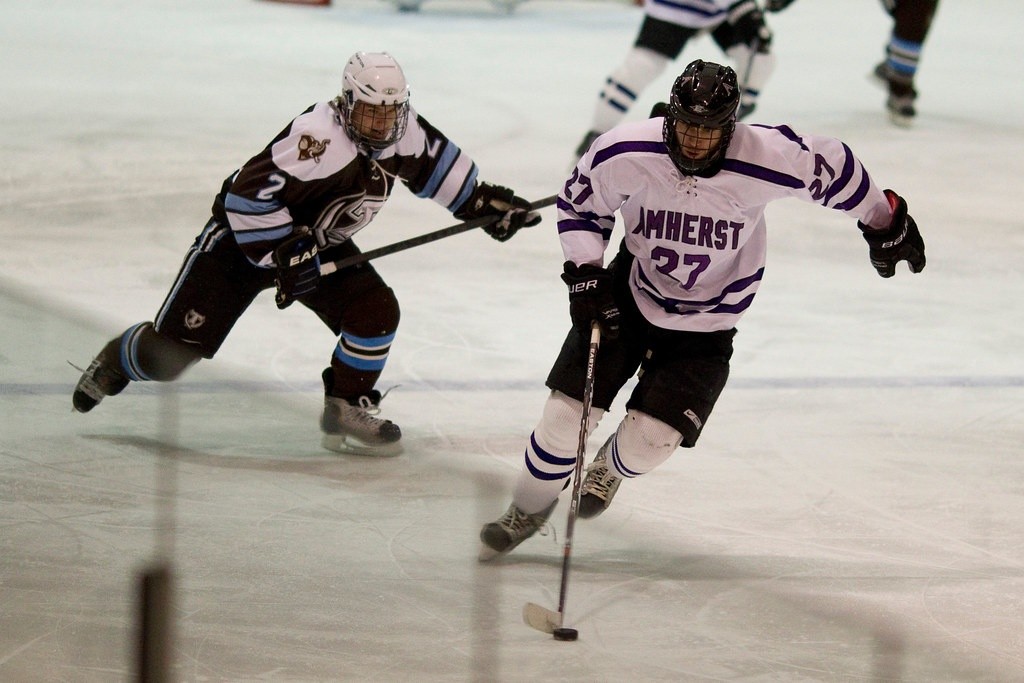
[521,319,602,636]
[734,0,772,121]
[318,191,562,277]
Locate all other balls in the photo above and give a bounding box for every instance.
[554,628,577,641]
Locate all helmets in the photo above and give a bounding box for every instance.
[667,59,741,173]
[340,51,411,151]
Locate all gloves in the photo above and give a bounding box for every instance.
[857,189,926,278]
[561,260,628,355]
[453,181,542,242]
[272,233,321,310]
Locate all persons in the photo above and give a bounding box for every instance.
[576,1,794,157]
[73,52,542,457]
[478,58,927,561]
[875,0,938,126]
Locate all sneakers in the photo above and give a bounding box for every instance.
[886,96,916,127]
[875,62,918,98]
[71,337,133,413]
[319,390,403,457]
[576,431,623,520]
[736,103,756,122]
[577,131,602,157]
[478,497,559,561]
